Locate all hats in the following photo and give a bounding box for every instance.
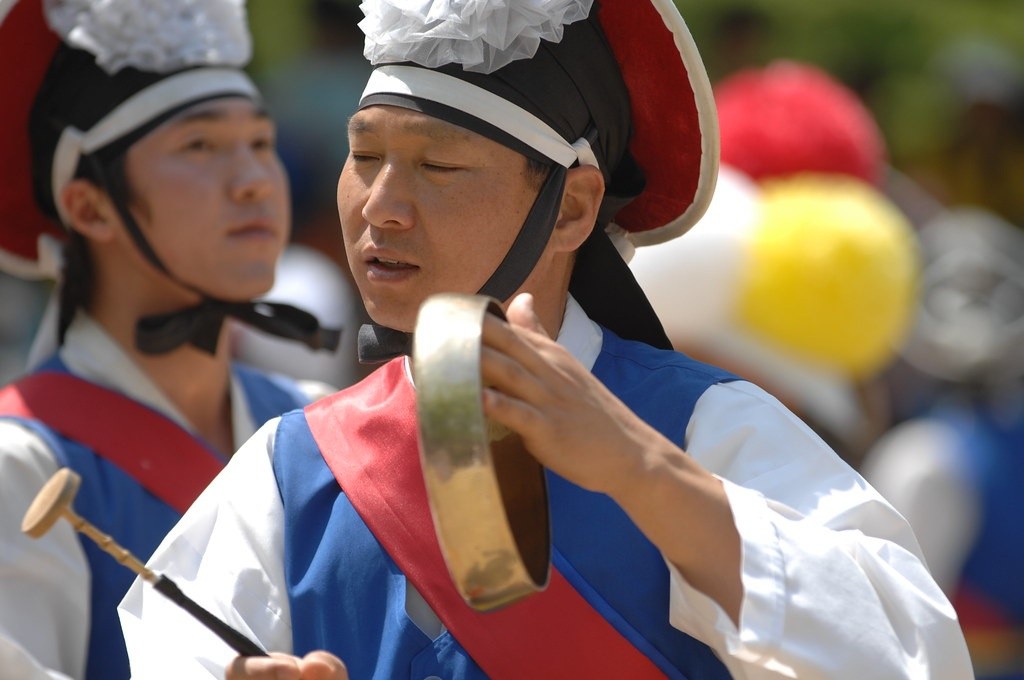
[354,0,719,264]
[0,0,267,282]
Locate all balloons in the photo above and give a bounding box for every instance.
[627,168,758,324]
[740,178,919,381]
[711,62,886,186]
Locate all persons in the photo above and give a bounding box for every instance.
[119,0,979,680]
[0,0,343,680]
[857,203,1024,680]
